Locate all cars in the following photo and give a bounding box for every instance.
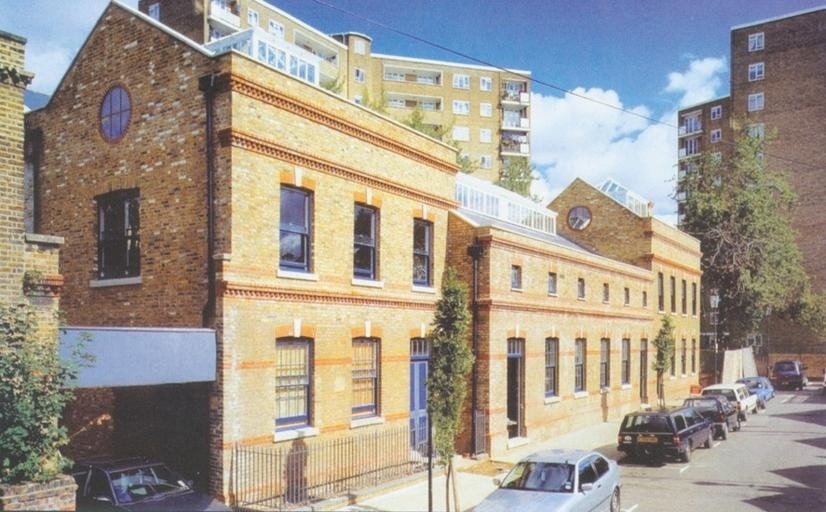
[472,449,623,512]
[64,454,235,512]
[617,359,807,462]
